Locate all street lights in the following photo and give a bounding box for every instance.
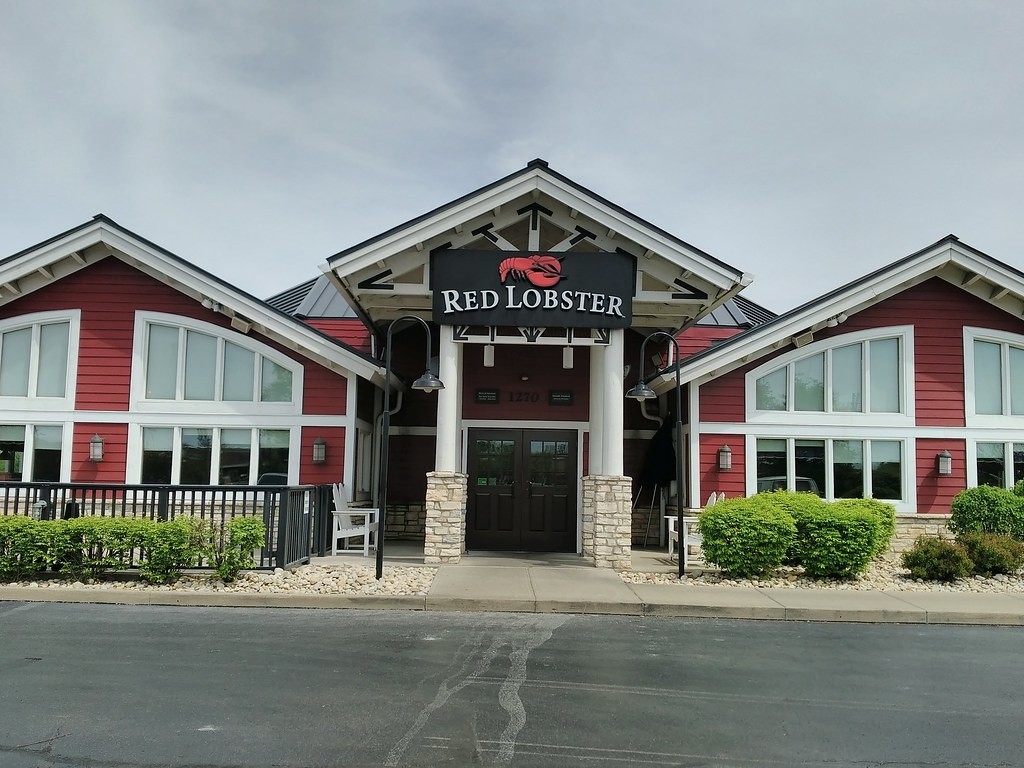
[624,331,685,580]
[376,314,445,579]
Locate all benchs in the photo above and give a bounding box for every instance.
[663,491,726,568]
[331,482,380,557]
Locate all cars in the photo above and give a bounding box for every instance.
[258,472,287,486]
[758,475,820,495]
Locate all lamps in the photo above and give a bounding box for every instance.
[625,384,657,402]
[88,432,104,463]
[937,448,952,476]
[411,374,445,393]
[312,436,327,464]
[719,443,732,472]
